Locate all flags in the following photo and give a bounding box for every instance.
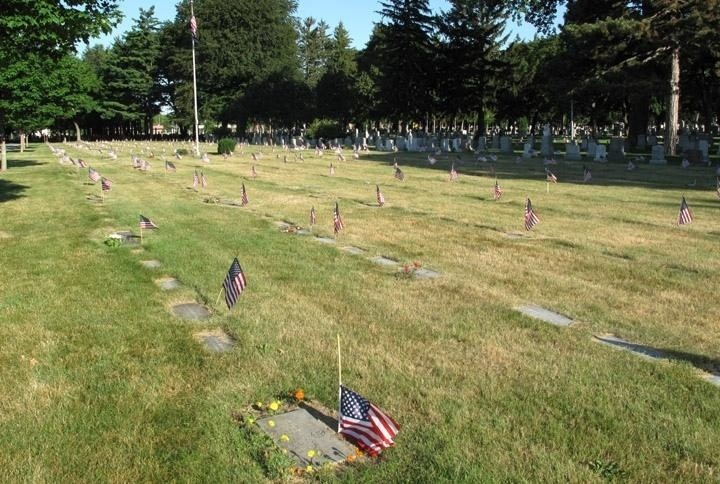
[220,257,247,310]
[189,4,199,43]
[376,144,504,209]
[547,166,592,184]
[525,201,539,230]
[53,138,364,207]
[677,197,692,225]
[140,215,158,229]
[310,205,344,232]
[338,383,402,458]
